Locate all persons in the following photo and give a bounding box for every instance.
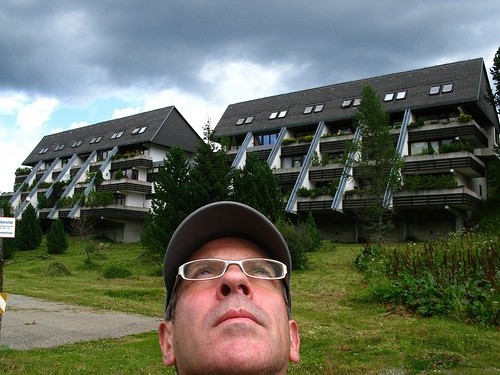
[159,200,300,375]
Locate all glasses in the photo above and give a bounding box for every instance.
[164,258,292,317]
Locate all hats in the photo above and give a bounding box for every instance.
[162,200,292,313]
